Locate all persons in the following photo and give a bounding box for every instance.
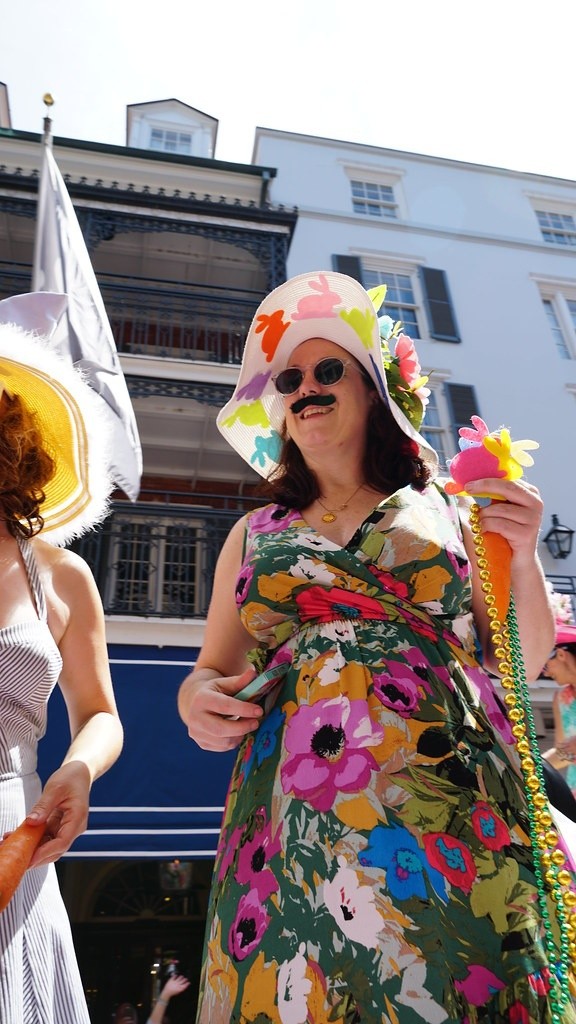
[176,272,575,1024]
[538,581,576,800]
[147,974,190,1024]
[0,292,144,1024]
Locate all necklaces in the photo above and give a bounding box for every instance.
[317,484,364,523]
[471,502,576,1023]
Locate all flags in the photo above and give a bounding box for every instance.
[32,144,142,503]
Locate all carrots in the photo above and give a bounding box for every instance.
[0,817,49,913]
[481,531,512,625]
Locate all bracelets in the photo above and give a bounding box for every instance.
[157,998,168,1006]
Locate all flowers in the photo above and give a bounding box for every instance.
[367,283,430,432]
[545,581,573,623]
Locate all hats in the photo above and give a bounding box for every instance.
[0,322,113,546]
[544,581,576,645]
[215,271,440,487]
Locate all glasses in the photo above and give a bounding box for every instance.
[271,357,366,397]
[542,646,568,671]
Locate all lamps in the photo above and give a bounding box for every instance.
[542,513,575,559]
